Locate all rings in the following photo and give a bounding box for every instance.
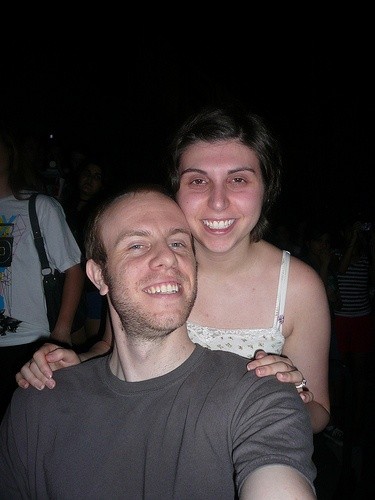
[296,376,308,390]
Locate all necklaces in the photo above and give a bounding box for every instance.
[80,198,88,202]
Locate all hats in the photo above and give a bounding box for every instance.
[79,209,97,226]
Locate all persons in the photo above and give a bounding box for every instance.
[0,189,317,500]
[0,136,375,436]
[16,112,332,434]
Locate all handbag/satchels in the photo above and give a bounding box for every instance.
[28,192,105,355]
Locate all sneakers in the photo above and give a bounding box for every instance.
[323,424,344,447]
